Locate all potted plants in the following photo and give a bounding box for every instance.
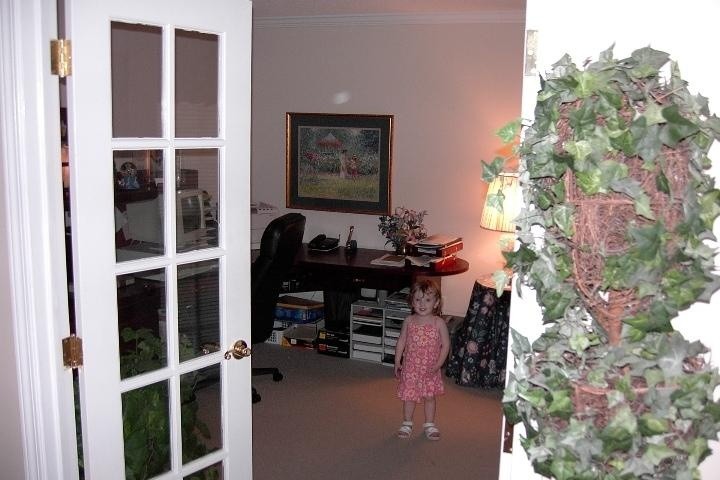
[478,42,720,480]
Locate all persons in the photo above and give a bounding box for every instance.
[340,148,358,180]
[394,278,451,442]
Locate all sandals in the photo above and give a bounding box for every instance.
[398,420,414,439]
[422,421,442,441]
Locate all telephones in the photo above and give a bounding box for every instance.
[307,234,340,251]
[120,161,139,188]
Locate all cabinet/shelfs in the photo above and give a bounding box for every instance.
[350,298,412,369]
[69,281,162,361]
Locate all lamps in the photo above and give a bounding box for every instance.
[480,171,520,286]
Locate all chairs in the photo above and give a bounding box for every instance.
[182,212,307,405]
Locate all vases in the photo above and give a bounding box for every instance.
[394,244,415,255]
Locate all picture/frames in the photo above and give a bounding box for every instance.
[284,112,394,218]
[143,143,182,193]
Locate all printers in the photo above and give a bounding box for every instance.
[250,203,279,249]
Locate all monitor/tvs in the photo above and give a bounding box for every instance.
[126,188,207,252]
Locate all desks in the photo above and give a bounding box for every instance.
[442,276,511,391]
[252,241,470,359]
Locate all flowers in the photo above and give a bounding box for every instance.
[377,205,430,248]
